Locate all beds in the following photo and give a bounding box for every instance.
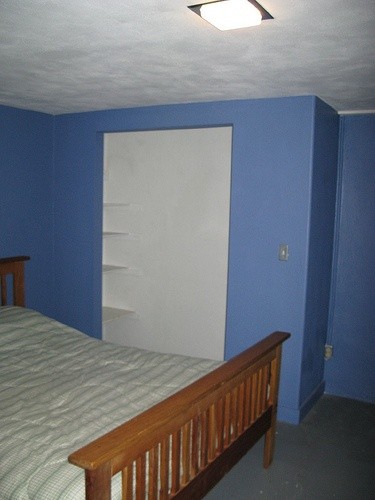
[1,303,276,499]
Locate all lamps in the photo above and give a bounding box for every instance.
[187,0,275,32]
